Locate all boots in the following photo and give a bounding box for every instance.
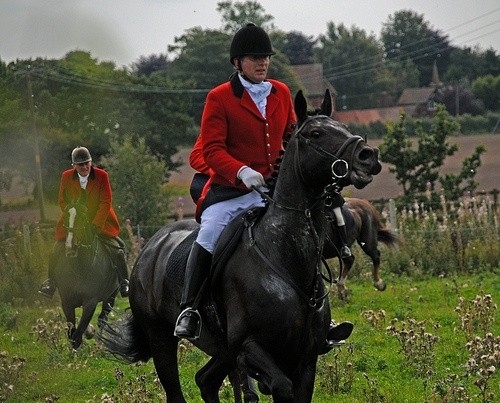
[175,239,212,336]
[316,278,353,356]
[116,260,130,296]
[40,253,57,298]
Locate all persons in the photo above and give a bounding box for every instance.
[173,22,354,356]
[38,146,132,297]
[188,130,211,204]
[326,186,353,259]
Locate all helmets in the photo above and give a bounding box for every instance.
[230,22,277,66]
[71,145,92,164]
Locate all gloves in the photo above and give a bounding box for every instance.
[237,166,266,190]
[91,224,100,235]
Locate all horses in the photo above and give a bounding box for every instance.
[93,87,383,403]
[48,187,130,350]
[322,196,398,300]
